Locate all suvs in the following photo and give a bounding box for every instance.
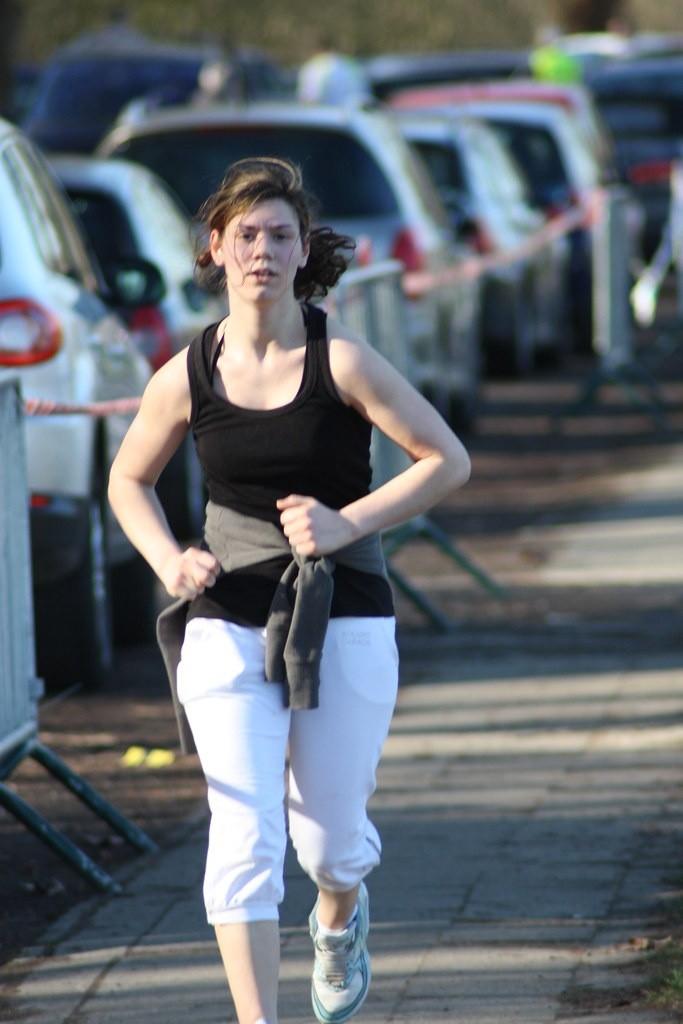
[0,122,177,688]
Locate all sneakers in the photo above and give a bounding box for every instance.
[309,881,372,1024]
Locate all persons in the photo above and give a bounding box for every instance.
[190,55,245,108]
[107,156,471,1024]
[526,27,587,86]
[297,28,374,111]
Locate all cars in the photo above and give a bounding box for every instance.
[37,147,232,537]
[12,30,679,379]
[84,100,486,452]
